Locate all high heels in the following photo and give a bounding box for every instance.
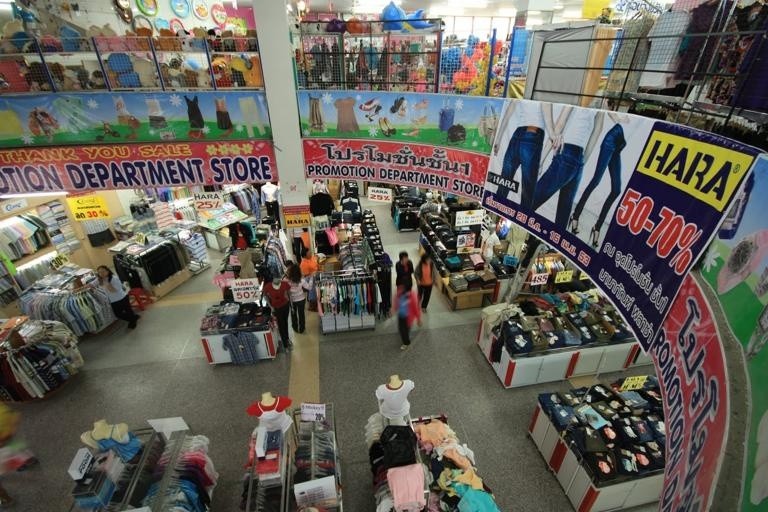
[567,216,579,234]
[589,224,600,247]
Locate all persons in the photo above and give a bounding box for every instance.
[566,111,647,249]
[310,192,335,217]
[0,401,38,471]
[522,105,605,229]
[97,265,140,329]
[260,248,434,354]
[261,182,282,229]
[81,419,143,462]
[290,228,310,263]
[244,391,294,434]
[480,222,501,263]
[416,192,438,218]
[227,222,254,250]
[375,374,415,419]
[506,222,529,261]
[493,98,558,210]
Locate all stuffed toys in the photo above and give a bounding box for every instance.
[0,19,264,90]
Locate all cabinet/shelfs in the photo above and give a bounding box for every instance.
[477,306,655,390]
[200,303,282,368]
[526,401,666,511]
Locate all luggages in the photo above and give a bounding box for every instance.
[439,97,453,131]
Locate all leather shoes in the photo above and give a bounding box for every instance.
[358,97,407,136]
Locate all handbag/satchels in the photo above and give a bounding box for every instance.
[446,124,467,145]
[477,104,499,136]
[127,115,139,128]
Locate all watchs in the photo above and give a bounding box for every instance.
[717,228,768,295]
[747,303,768,355]
[718,171,755,239]
[754,264,768,297]
